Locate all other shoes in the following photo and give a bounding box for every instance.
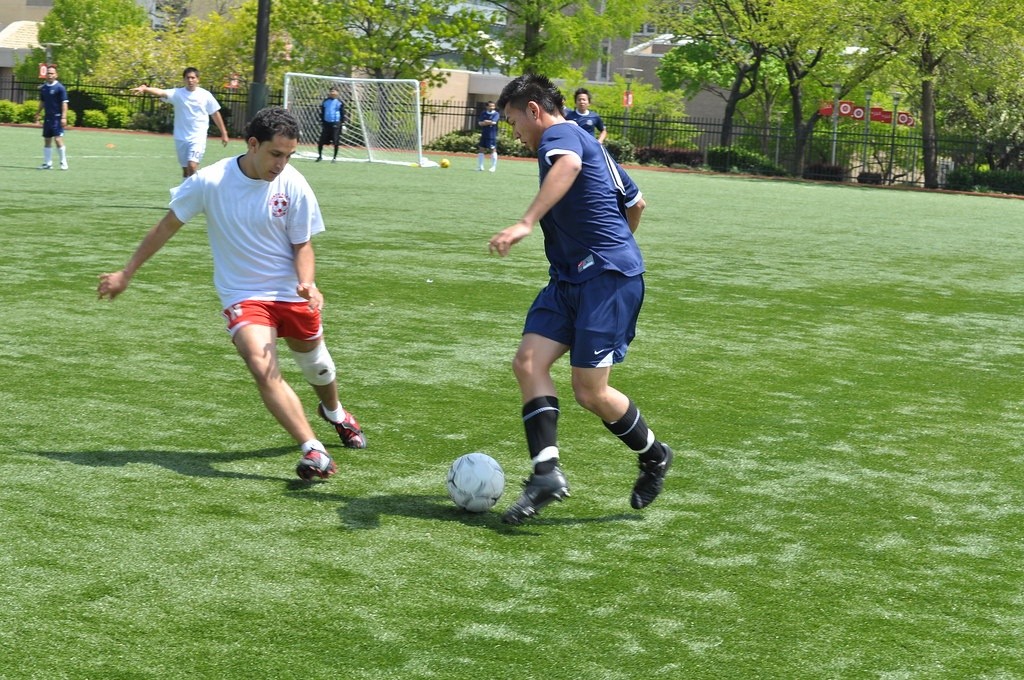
[36,163,53,170]
[331,160,335,163]
[61,161,68,170]
[316,157,322,162]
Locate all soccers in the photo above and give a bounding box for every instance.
[447,451,506,513]
[440,159,449,168]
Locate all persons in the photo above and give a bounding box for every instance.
[97,107,365,482]
[488,72,672,523]
[315,86,346,163]
[130,67,229,182]
[35,66,68,170]
[478,101,500,172]
[561,88,606,142]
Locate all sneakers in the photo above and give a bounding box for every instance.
[297,449,336,480]
[504,467,570,525]
[631,442,672,510]
[318,402,366,449]
